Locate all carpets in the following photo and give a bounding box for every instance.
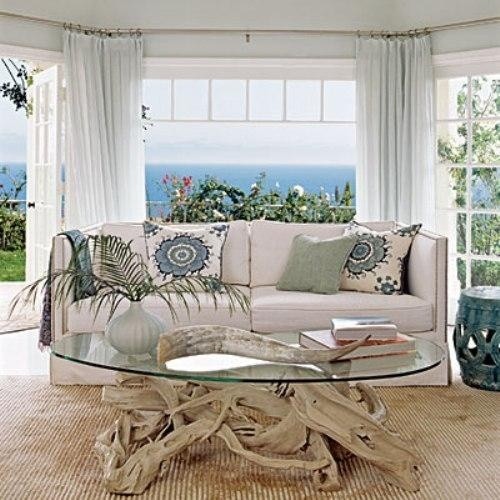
[1,372,500,499]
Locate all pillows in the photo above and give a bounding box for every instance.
[142,220,229,292]
[275,219,422,295]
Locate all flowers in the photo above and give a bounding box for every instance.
[156,174,336,224]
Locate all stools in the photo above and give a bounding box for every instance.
[453,286,500,392]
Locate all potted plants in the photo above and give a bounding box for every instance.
[8,235,252,356]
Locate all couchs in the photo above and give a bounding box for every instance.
[50,220,450,388]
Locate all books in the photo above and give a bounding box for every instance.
[298,317,422,375]
